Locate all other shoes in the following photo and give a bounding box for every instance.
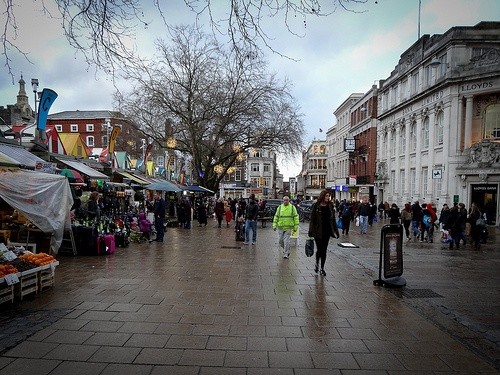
[243,242,249,245]
[283,250,290,259]
[314,265,319,273]
[252,240,255,245]
[320,268,326,276]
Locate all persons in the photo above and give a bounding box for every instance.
[308,191,339,276]
[148,193,165,242]
[294,196,304,205]
[273,196,299,259]
[243,194,259,245]
[333,196,487,248]
[88,191,100,219]
[177,195,248,229]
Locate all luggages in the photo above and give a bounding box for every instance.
[97,230,114,256]
[115,231,129,248]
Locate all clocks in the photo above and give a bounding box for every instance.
[344,139,356,150]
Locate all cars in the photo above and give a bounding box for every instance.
[239,197,316,224]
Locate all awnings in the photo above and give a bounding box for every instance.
[120,168,213,193]
[0,143,47,168]
[54,157,110,178]
[107,181,140,187]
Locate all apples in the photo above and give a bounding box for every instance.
[0,264,18,278]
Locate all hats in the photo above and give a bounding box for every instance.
[249,194,255,200]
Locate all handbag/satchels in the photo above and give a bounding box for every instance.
[305,237,314,257]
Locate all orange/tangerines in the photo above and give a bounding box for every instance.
[19,252,55,266]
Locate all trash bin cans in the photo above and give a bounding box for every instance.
[235,220,246,241]
